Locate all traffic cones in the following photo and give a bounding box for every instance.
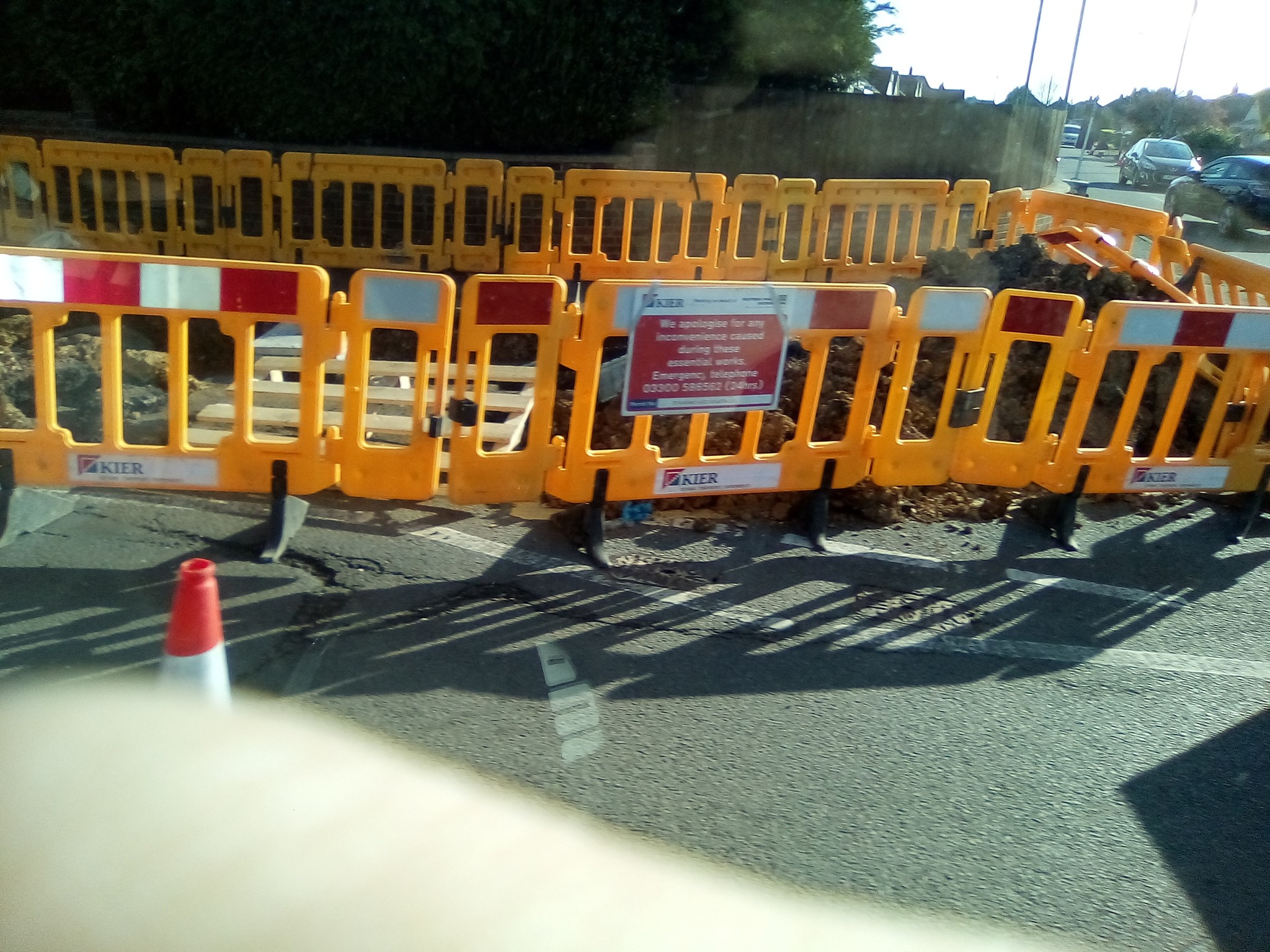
[152,556,236,704]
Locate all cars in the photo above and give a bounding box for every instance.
[1118,138,1202,190]
[1061,124,1081,146]
[1163,154,1270,240]
